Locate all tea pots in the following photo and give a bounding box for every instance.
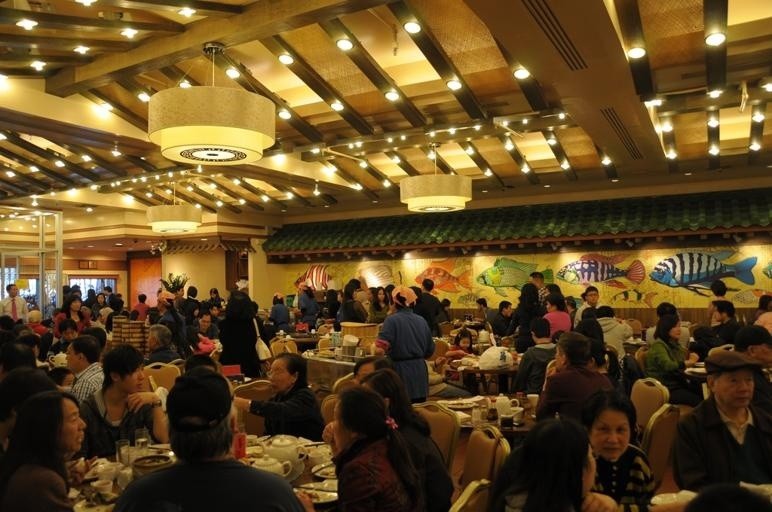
[48,351,68,368]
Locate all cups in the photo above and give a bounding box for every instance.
[286,335,291,339]
[494,392,539,431]
[343,334,359,347]
[342,345,357,362]
[311,329,315,334]
[479,331,489,342]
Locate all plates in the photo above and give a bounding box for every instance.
[68,432,341,511]
[650,492,679,505]
[686,362,708,374]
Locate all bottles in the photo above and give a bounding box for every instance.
[510,347,519,365]
[470,397,499,428]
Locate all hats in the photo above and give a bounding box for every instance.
[733,324,771,349]
[703,345,763,376]
[552,330,594,359]
[164,365,233,433]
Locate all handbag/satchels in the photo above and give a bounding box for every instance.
[255,337,273,361]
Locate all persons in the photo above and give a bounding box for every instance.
[0,270,771,511]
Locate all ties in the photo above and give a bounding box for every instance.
[11,298,19,321]
